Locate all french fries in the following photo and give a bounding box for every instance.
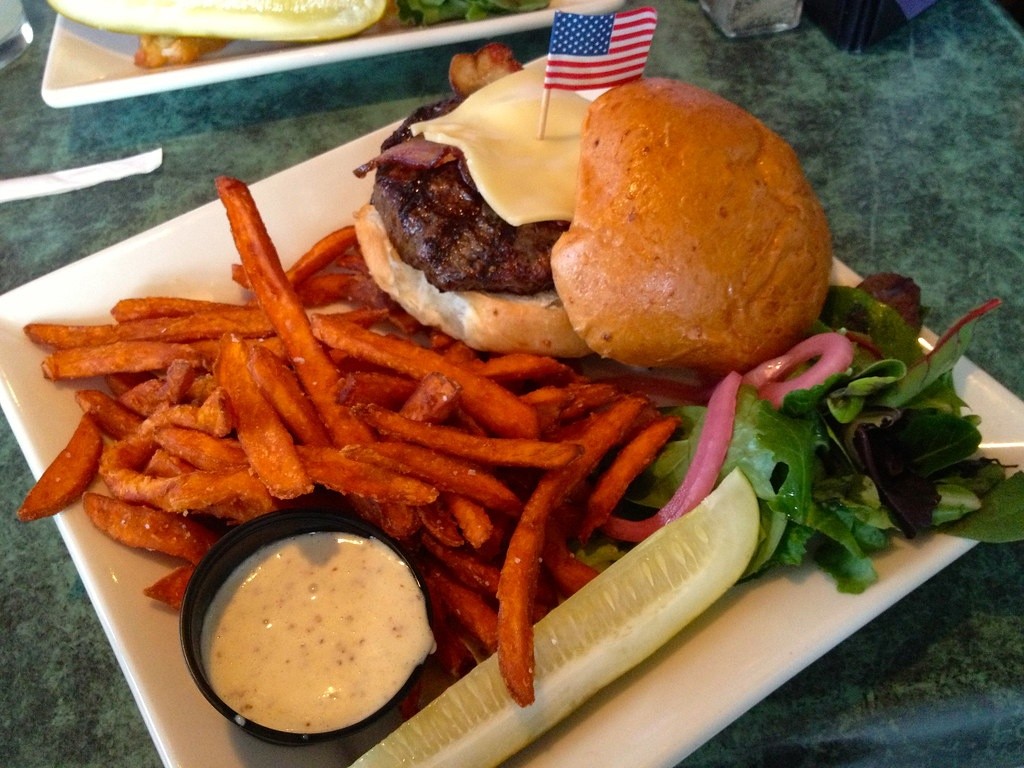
[15,175,684,707]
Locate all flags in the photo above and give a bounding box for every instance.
[545,8,659,89]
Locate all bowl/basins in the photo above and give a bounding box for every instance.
[178,502,433,747]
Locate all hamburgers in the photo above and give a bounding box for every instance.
[352,36,834,375]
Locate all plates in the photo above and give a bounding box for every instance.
[0,56,1022,768]
[40,0,636,107]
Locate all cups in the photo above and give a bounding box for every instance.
[700,0,803,40]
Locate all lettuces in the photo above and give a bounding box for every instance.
[634,272,1023,593]
